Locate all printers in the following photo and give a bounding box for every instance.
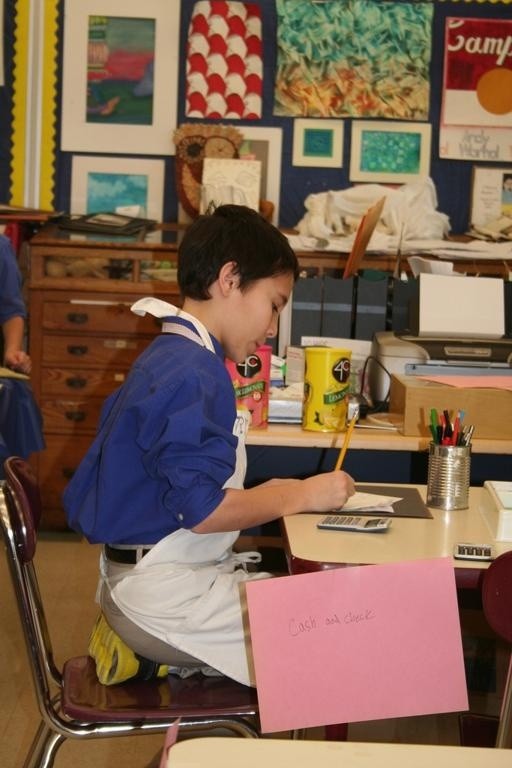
[369,272,512,411]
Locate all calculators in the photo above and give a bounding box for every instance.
[317,516,392,532]
[454,544,496,561]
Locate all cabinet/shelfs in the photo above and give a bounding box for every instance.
[24,236,191,532]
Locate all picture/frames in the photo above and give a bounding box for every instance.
[223,125,284,230]
[348,118,433,186]
[288,115,344,171]
[59,0,183,158]
[67,155,167,247]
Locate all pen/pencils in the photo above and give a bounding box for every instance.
[429,408,476,447]
[334,407,360,472]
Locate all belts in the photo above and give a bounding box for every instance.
[104,543,150,564]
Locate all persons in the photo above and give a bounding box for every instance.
[63,202,359,688]
[0,230,48,475]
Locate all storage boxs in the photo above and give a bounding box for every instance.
[386,371,512,440]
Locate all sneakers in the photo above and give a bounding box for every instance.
[88,610,169,687]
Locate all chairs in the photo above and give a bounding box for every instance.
[0,448,258,767]
[472,547,511,750]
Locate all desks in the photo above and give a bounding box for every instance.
[286,241,512,340]
[242,409,511,456]
[281,477,512,570]
